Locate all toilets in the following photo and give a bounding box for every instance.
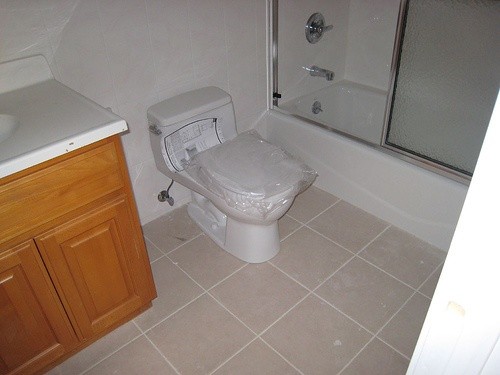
[146,86,303,264]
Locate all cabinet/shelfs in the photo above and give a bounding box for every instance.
[1,115,159,372]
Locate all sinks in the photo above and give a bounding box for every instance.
[0,113,19,142]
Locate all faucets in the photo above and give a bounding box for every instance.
[310,65,334,80]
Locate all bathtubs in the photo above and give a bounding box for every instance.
[269,79,485,240]
